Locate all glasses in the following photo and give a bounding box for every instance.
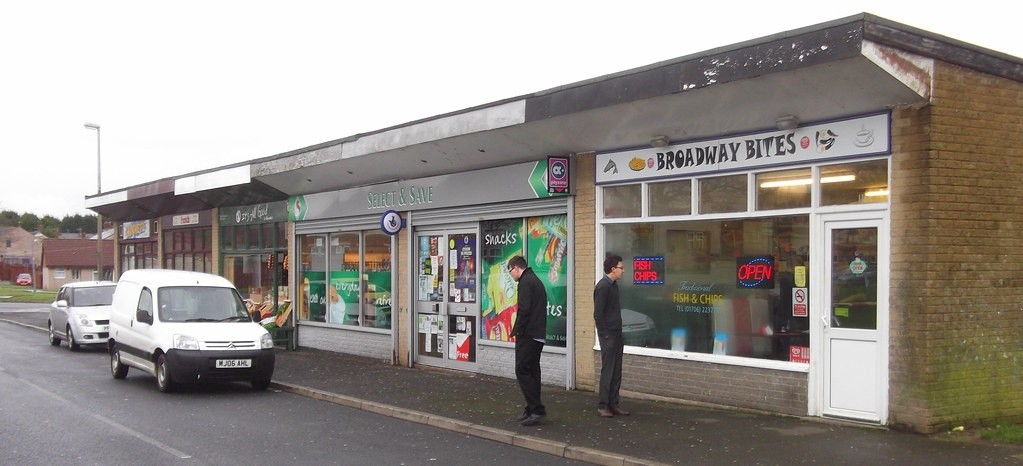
[508,268,515,275]
[611,266,624,270]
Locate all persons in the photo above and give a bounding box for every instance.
[780,256,808,353]
[506,256,548,426]
[594,254,629,418]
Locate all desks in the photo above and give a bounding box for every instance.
[737,330,802,361]
[267,326,294,352]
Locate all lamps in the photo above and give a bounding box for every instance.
[774,116,798,130]
[865,187,888,197]
[650,136,669,147]
[759,169,857,189]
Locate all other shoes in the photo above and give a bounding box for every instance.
[522,413,547,426]
[597,408,614,417]
[611,406,630,415]
[517,406,531,420]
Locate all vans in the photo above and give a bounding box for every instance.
[107,269,276,394]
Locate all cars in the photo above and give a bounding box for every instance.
[15,273,32,286]
[48,280,118,351]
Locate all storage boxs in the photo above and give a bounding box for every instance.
[247,294,292,327]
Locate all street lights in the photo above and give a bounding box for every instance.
[84,122,106,280]
